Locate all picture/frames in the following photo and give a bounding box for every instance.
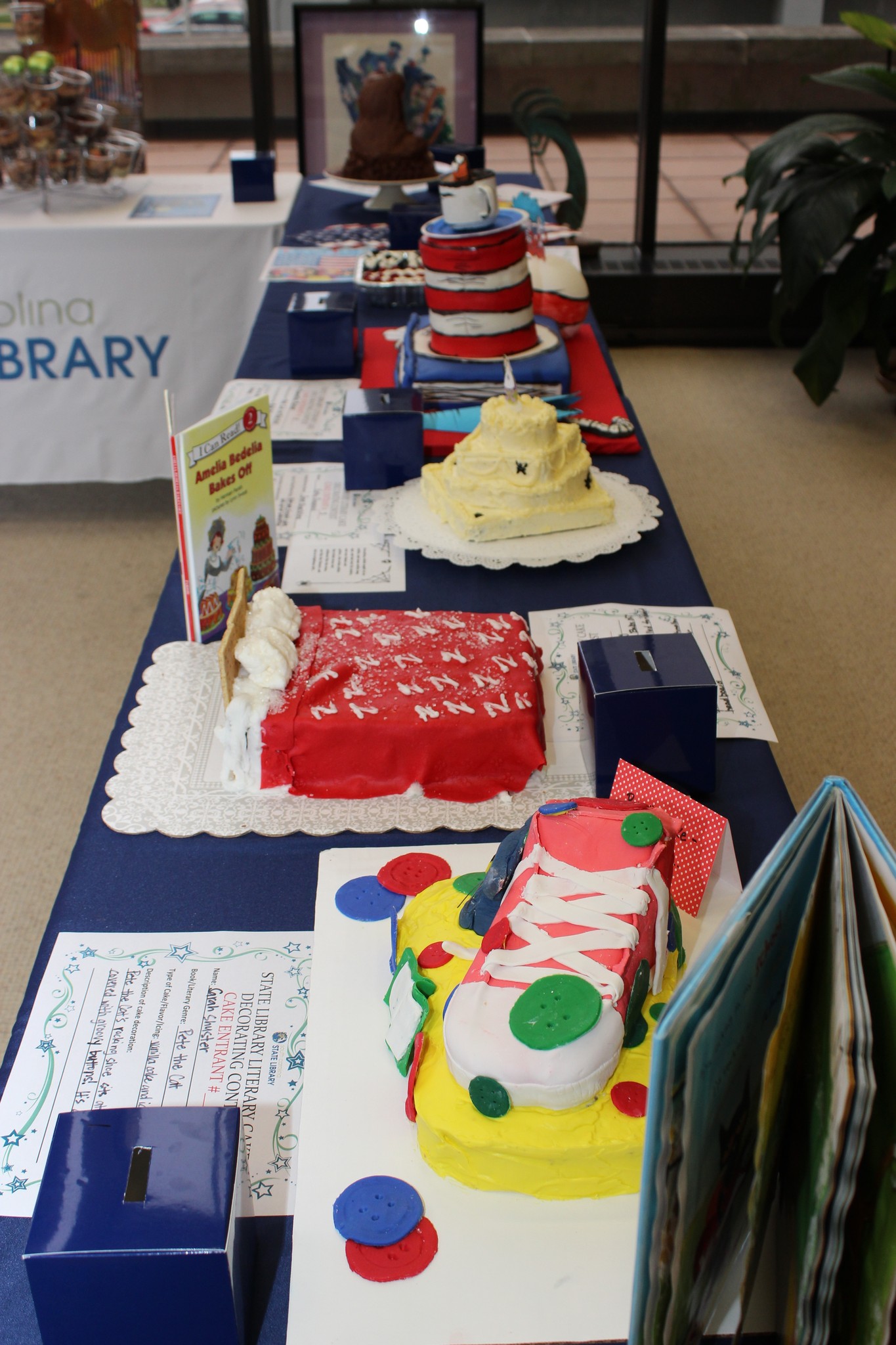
[289,0,490,185]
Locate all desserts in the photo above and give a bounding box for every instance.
[0,4,149,187]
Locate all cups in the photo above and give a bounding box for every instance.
[435,168,499,230]
[0,3,149,186]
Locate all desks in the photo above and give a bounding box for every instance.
[0,170,896,1345]
[0,165,308,499]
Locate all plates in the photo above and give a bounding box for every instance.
[420,206,530,239]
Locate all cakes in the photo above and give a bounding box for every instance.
[373,756,727,1201]
[417,393,615,542]
[418,169,537,359]
[340,71,436,180]
[211,567,546,801]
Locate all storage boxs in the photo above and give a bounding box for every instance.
[382,200,441,252]
[336,383,430,494]
[16,1101,249,1345]
[562,626,724,813]
[282,283,366,378]
[225,147,284,208]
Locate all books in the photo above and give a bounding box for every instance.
[164,388,282,644]
[632,772,896,1344]
